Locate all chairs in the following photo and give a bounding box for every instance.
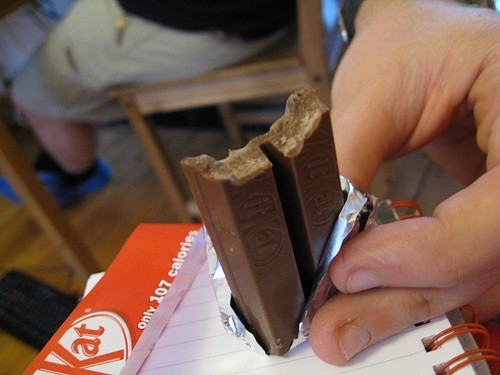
[106,0,333,225]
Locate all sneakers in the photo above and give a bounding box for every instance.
[1,147,112,211]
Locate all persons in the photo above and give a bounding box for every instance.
[307,0,499,368]
[9,1,299,215]
